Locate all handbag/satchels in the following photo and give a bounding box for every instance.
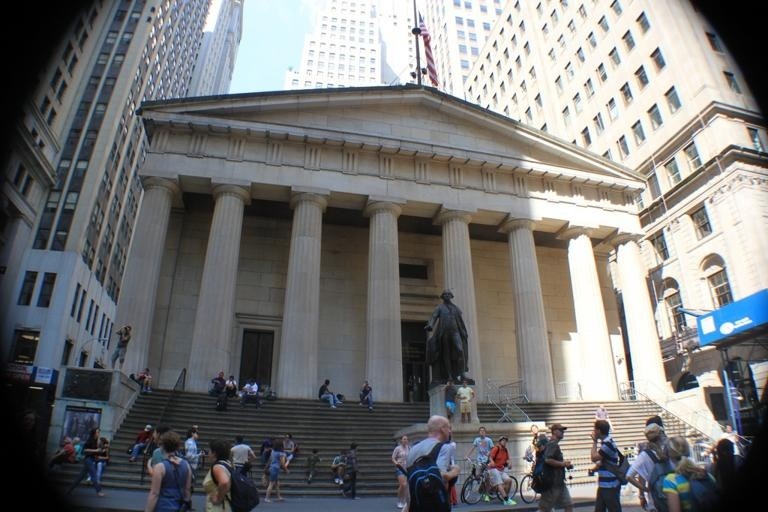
[616,455,631,485]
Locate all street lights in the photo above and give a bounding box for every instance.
[71,338,108,367]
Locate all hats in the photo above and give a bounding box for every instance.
[552,424,566,431]
[644,423,662,441]
[351,443,358,449]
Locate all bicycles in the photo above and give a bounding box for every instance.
[458,458,536,505]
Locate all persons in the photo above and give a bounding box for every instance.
[83,428,111,498]
[443,383,475,424]
[129,422,358,510]
[209,372,260,409]
[423,287,474,381]
[358,379,373,410]
[106,321,132,372]
[392,405,766,511]
[139,369,153,393]
[318,379,343,410]
[52,435,84,463]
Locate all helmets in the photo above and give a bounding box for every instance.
[499,436,508,443]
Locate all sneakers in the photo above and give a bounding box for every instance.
[335,478,339,483]
[503,498,517,505]
[485,495,489,502]
[397,501,404,508]
[480,493,485,502]
[340,479,343,484]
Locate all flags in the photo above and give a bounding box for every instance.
[414,10,439,91]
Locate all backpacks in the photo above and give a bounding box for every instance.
[405,443,452,512]
[691,471,714,512]
[644,447,675,512]
[532,450,555,493]
[211,461,260,512]
